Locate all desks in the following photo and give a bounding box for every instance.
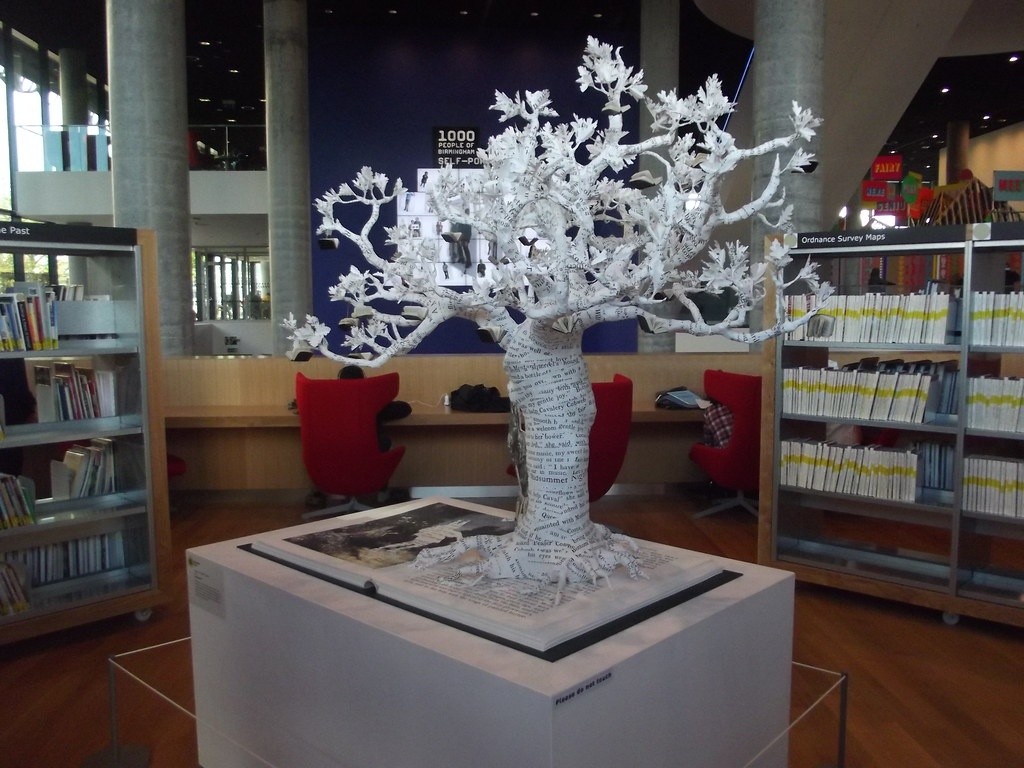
[165,404,704,428]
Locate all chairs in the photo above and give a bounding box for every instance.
[505,374,634,503]
[688,369,763,519]
[295,371,405,519]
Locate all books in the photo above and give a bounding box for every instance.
[781,281,1024,520]
[0,279,126,618]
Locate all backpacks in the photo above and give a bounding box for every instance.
[451,383,510,413]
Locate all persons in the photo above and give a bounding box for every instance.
[694,399,734,500]
[305,365,414,509]
[241,292,270,321]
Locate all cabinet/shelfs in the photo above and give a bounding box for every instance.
[0,220,177,646]
[756,220,1024,626]
